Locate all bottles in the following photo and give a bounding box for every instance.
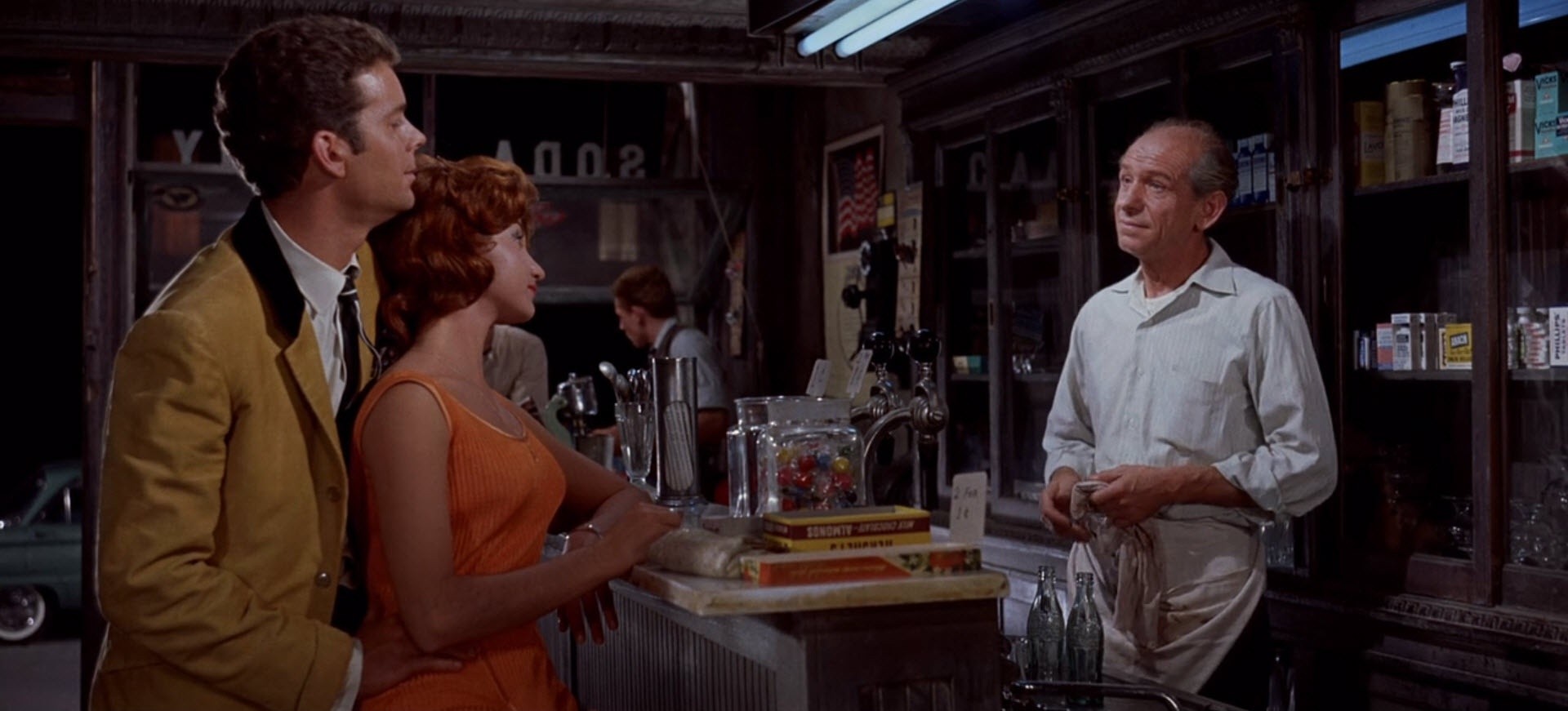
[1064,572,1103,710]
[729,392,832,519]
[756,399,869,520]
[1026,567,1063,684]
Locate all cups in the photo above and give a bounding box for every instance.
[616,399,655,483]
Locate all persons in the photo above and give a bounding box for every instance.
[610,265,738,505]
[353,152,684,711]
[482,322,548,414]
[1038,120,1336,710]
[86,17,426,711]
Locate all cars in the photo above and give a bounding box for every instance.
[0,463,87,646]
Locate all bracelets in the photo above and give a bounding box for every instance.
[563,525,604,539]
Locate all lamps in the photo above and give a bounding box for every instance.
[745,0,969,77]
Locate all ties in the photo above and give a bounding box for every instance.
[333,272,362,470]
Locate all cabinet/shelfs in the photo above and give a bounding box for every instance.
[1314,0,1567,616]
[927,73,1064,519]
[1092,47,1298,301]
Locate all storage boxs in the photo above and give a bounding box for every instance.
[738,541,984,590]
[761,503,932,555]
